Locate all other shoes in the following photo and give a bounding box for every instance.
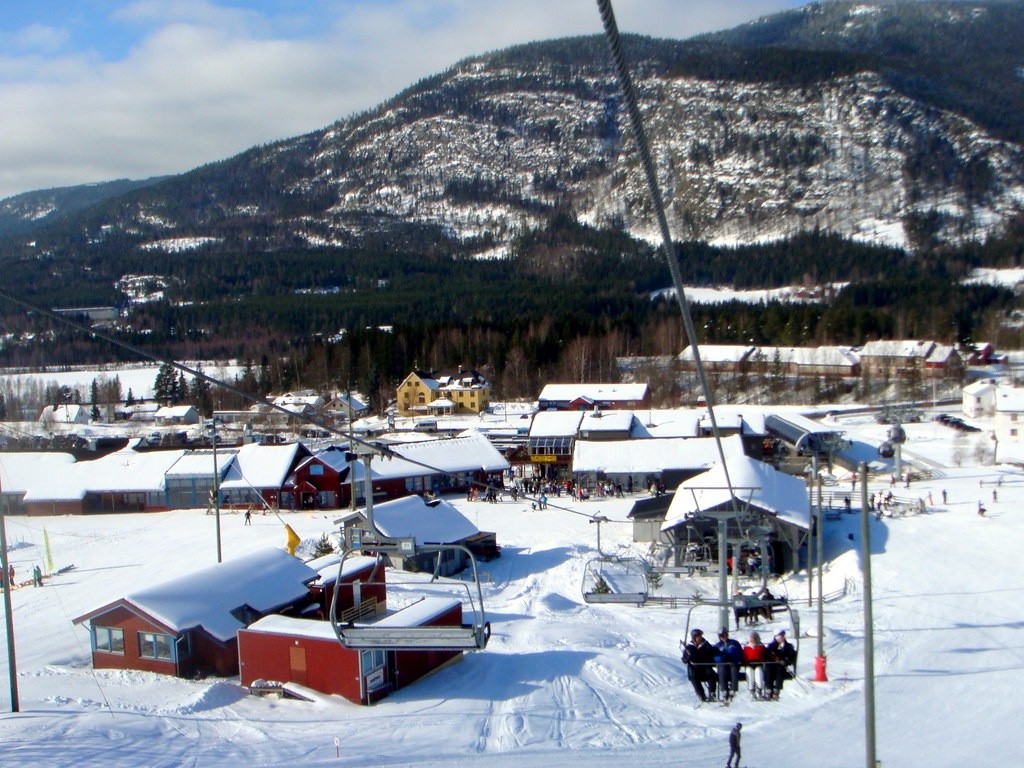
[700,686,781,703]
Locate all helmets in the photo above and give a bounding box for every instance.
[716,627,728,635]
[691,629,703,645]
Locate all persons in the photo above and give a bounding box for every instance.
[735,588,775,631]
[261,501,268,515]
[727,551,771,577]
[869,468,1005,521]
[8,565,15,586]
[244,510,251,526]
[35,565,43,587]
[725,723,742,768]
[681,626,795,702]
[0,567,3,588]
[303,494,314,510]
[851,472,857,491]
[465,465,665,512]
[845,496,852,514]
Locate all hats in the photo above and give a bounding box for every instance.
[751,631,761,639]
[773,628,785,637]
